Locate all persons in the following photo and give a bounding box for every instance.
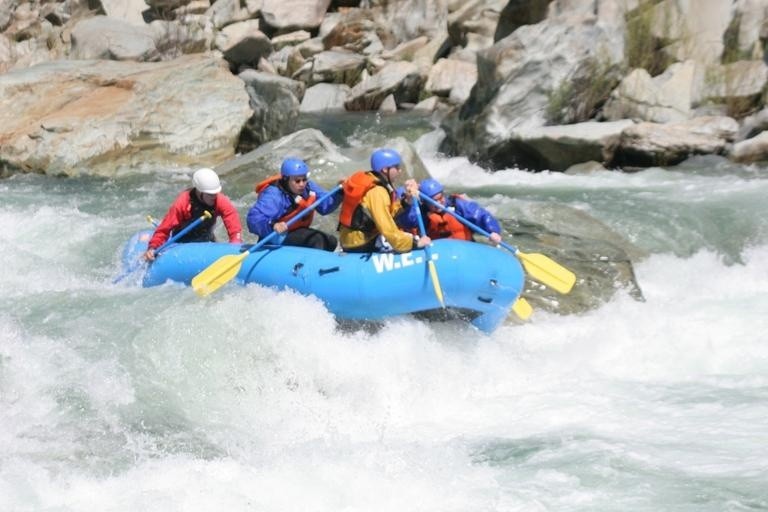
[247,157,347,252]
[336,147,501,255]
[146,168,243,261]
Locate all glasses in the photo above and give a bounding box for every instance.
[294,179,307,183]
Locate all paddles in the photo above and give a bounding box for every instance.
[407,186,576,294]
[191,184,343,297]
[512,297,532,321]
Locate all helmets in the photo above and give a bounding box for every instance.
[372,149,400,171]
[281,158,309,177]
[192,168,222,195]
[420,178,443,203]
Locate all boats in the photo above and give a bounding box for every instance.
[120,230,529,337]
